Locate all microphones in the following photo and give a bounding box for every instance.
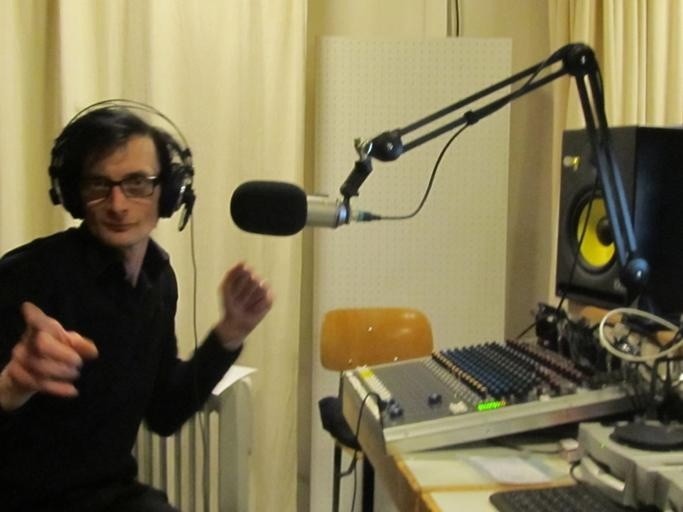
[230,180,349,236]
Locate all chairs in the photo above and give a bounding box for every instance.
[319,308,434,512]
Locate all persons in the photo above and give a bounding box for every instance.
[0,98,277,511]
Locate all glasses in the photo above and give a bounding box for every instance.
[75,176,161,200]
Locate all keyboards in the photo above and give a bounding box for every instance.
[488,483,635,512]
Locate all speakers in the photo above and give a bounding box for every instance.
[556,125,683,312]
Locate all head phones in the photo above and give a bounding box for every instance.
[49,98,196,231]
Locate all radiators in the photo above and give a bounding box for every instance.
[132,377,251,512]
